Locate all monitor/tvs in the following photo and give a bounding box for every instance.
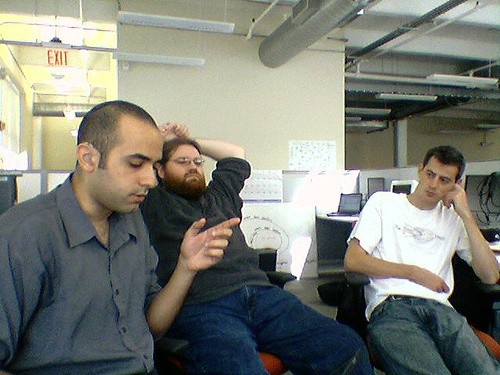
[464,174,500,229]
[391,180,419,195]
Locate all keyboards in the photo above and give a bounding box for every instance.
[480,229,500,242]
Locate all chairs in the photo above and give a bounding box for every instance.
[336,271,500,359]
[157,272,297,374]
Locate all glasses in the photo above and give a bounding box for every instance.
[167,156,205,167]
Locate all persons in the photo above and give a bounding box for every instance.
[139,122,375,375]
[343,145,500,375]
[0,100,240,375]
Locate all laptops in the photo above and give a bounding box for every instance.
[327,193,362,217]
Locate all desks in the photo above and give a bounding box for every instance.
[317,214,359,223]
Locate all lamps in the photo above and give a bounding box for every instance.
[0,0,500,129]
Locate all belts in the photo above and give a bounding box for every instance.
[372,295,417,317]
